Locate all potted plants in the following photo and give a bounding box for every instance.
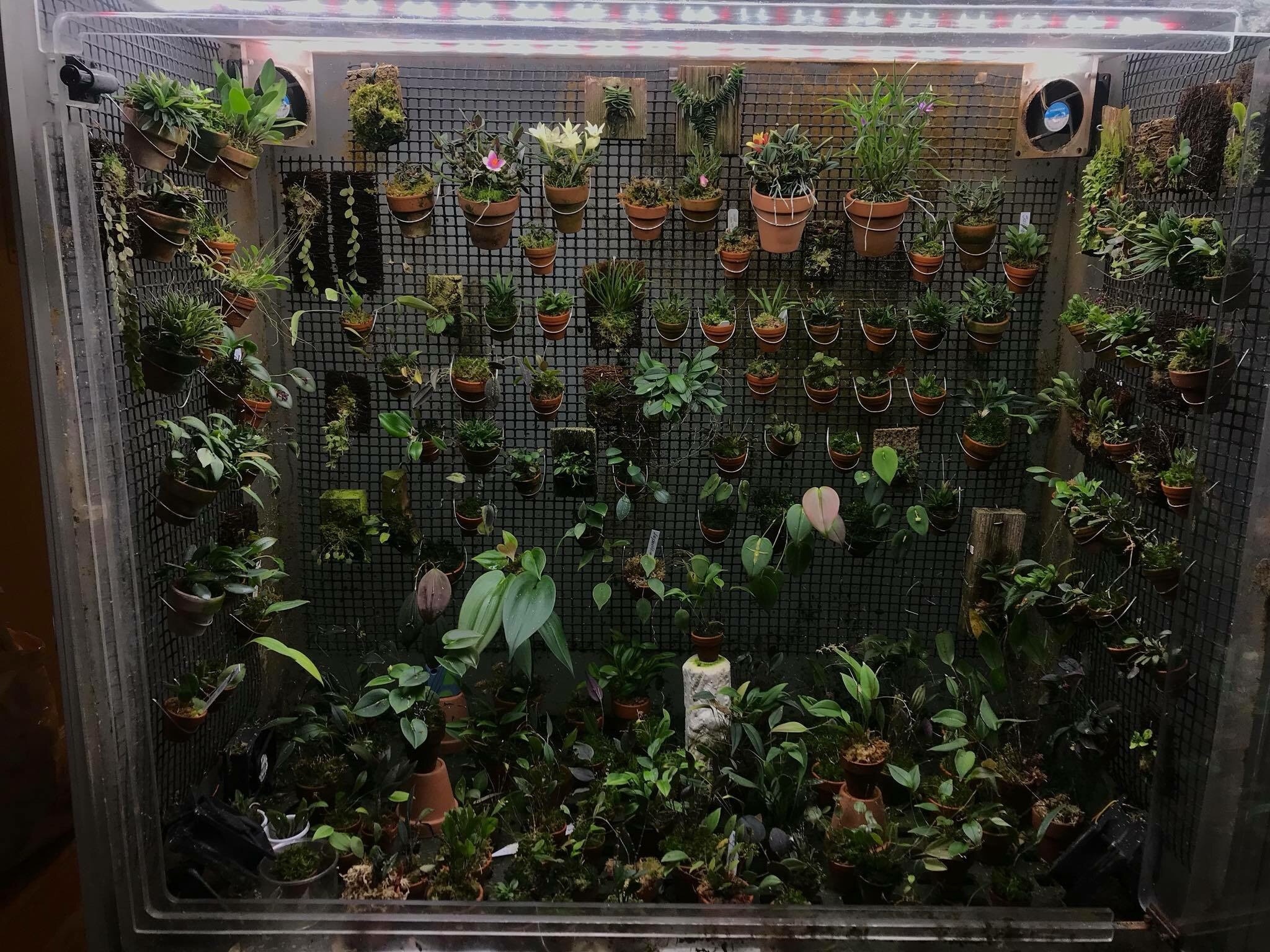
[90,60,1265,904]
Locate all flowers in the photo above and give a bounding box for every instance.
[526,118,605,187]
[738,121,839,225]
[678,142,725,200]
[428,108,540,203]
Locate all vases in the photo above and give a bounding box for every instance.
[456,185,520,250]
[545,177,589,233]
[680,192,724,231]
[751,183,815,254]
